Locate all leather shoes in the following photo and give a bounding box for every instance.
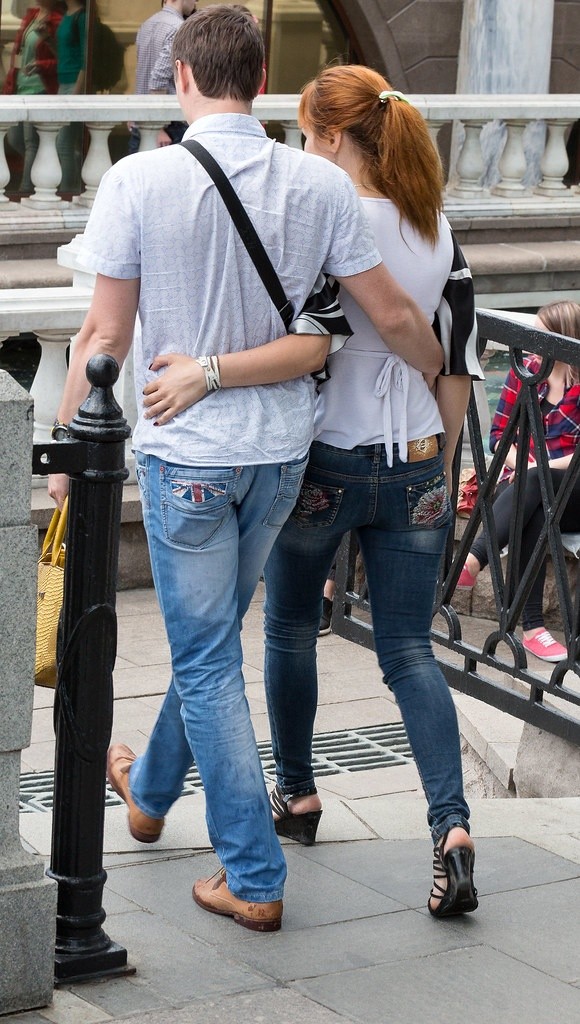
[105,743,164,844]
[193,867,282,932]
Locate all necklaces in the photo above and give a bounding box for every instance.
[354,183,374,187]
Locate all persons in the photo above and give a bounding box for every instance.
[317,534,361,636]
[142,66,485,919]
[127,0,199,154]
[47,4,446,933]
[437,300,580,662]
[0,0,102,192]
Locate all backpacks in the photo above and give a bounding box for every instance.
[71,10,123,89]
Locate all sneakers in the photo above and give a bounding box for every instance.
[319,595,334,636]
[436,563,476,591]
[522,627,568,662]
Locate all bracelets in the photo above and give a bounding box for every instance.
[198,356,222,391]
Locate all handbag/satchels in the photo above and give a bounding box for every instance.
[458,464,478,520]
[2,48,22,95]
[34,495,68,689]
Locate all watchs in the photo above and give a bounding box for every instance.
[51,418,70,441]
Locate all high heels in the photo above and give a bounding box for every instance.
[268,785,322,847]
[427,824,478,918]
